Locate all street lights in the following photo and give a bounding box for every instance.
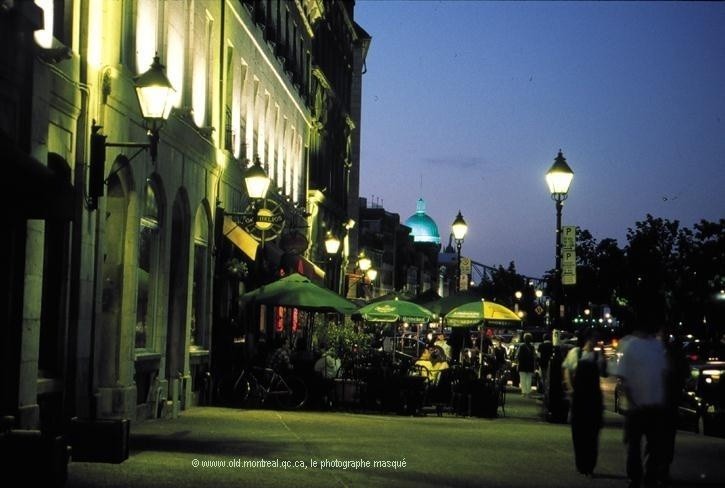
[451,209,468,295]
[545,150,573,421]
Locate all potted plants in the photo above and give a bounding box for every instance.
[325,322,372,401]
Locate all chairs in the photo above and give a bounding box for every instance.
[382,364,512,417]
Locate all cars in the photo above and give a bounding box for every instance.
[590,333,725,436]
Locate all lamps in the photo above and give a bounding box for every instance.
[87,51,185,206]
[214,156,270,253]
[306,229,341,262]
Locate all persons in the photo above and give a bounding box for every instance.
[495,310,678,488]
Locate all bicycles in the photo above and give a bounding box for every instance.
[216,363,308,410]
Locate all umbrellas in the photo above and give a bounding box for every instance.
[231,272,521,380]
